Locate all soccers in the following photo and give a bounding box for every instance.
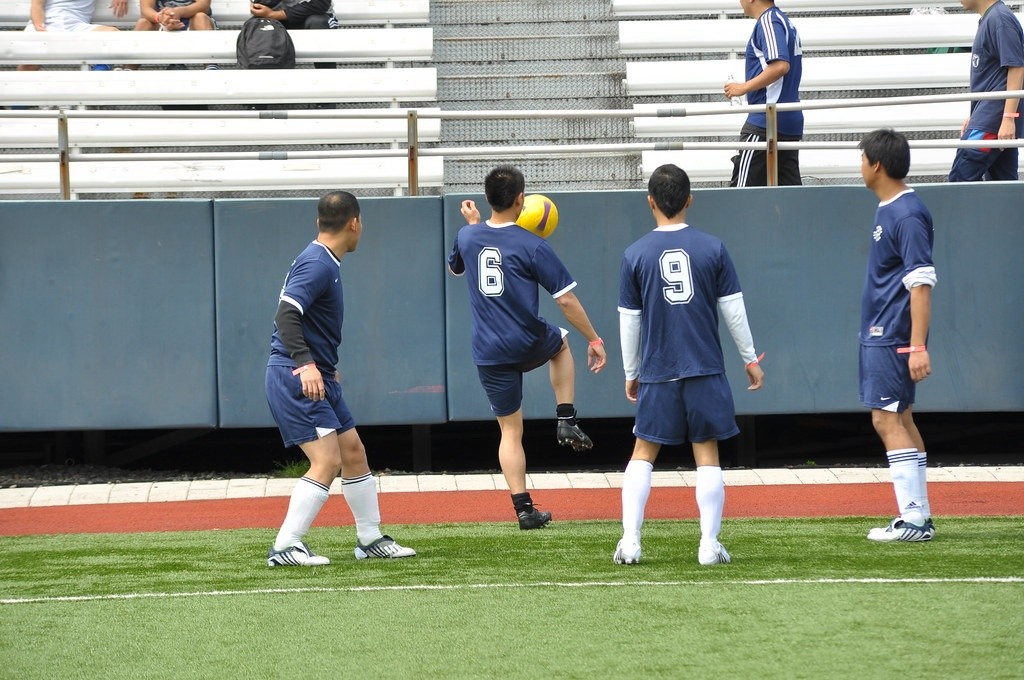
[516,195,559,240]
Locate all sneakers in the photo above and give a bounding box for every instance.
[698,541,731,566]
[354,533,416,559]
[866,515,935,542]
[267,540,330,566]
[613,540,642,566]
[518,507,551,530]
[557,418,593,451]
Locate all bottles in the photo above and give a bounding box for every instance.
[727,74,742,107]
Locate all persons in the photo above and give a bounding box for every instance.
[854,128,938,543]
[612,163,764,567]
[9,0,121,110]
[250,0,338,108]
[948,0,1024,182]
[123,0,221,70]
[722,0,804,187]
[447,164,607,531]
[264,191,417,566]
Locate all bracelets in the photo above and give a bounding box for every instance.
[292,363,315,376]
[897,345,926,354]
[589,337,604,346]
[1004,113,1020,118]
[748,352,765,366]
[155,12,160,22]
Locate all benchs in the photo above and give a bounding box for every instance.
[1,0,1024,199]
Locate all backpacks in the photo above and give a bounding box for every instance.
[235,15,295,70]
